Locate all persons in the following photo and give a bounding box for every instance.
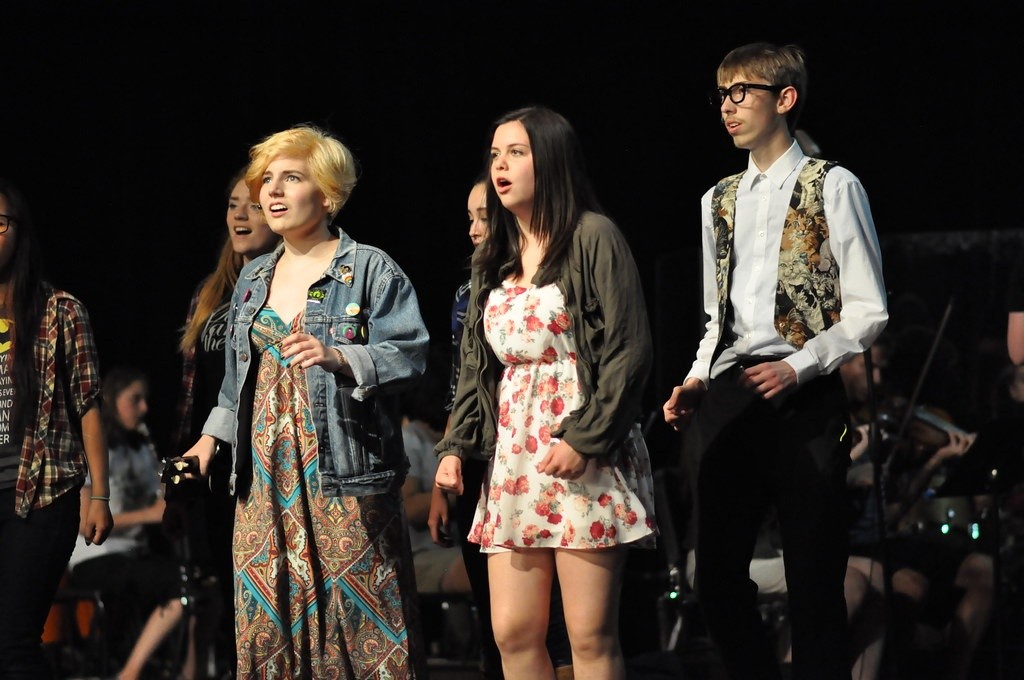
[440,172,572,680]
[661,40,891,680]
[70,263,1024,680]
[431,107,658,679]
[171,170,282,680]
[0,191,113,679]
[173,129,431,680]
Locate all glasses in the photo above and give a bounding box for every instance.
[0,214,20,234]
[861,359,889,373]
[709,82,786,109]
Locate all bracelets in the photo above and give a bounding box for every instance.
[90,496,110,502]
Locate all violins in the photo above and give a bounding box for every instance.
[858,389,974,452]
